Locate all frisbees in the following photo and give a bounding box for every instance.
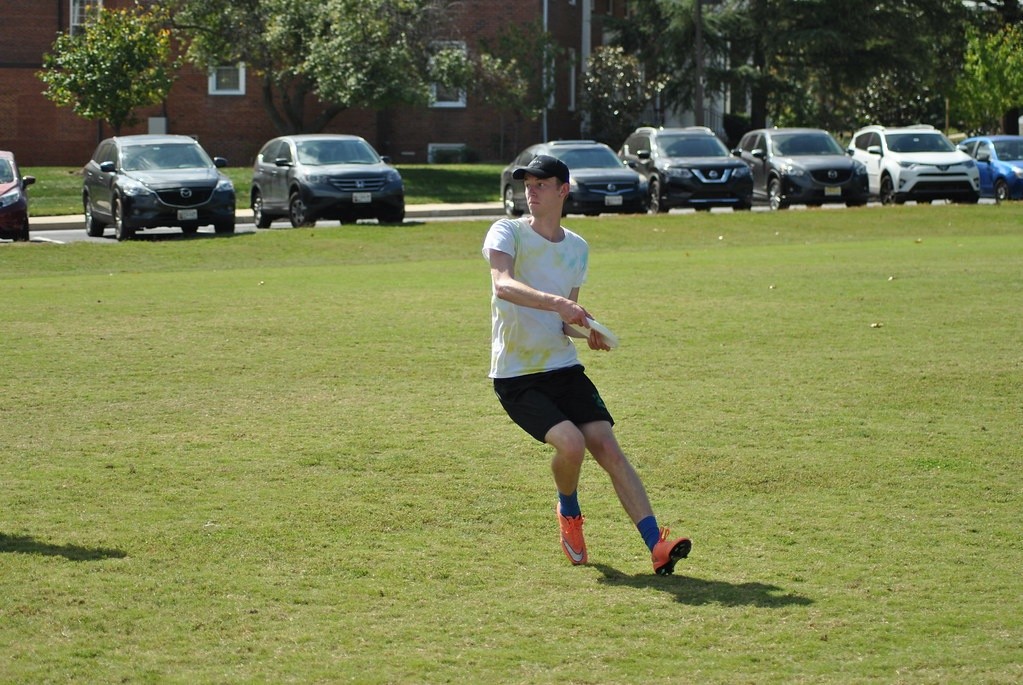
[568,315,618,350]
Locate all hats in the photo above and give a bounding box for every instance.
[513,155,569,185]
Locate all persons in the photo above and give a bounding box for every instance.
[481,155,693,578]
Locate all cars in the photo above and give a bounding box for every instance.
[499,138,650,220]
[957,133,1023,203]
[730,128,870,210]
[249,131,407,229]
[0,149,36,244]
[81,134,236,243]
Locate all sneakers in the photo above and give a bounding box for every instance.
[556,501,588,565]
[651,525,691,576]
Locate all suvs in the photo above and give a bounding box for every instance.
[616,125,754,215]
[843,123,981,205]
